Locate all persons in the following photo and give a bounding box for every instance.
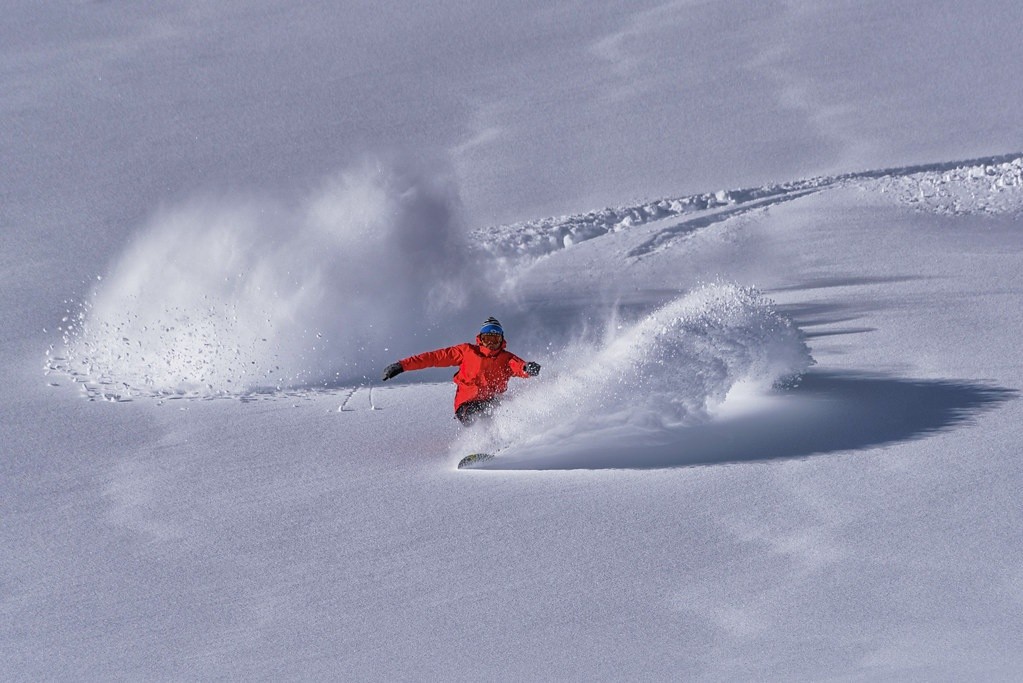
[382,316,540,428]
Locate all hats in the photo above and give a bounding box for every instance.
[481,317,503,334]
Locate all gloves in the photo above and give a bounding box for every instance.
[526,362,541,377]
[382,362,404,382]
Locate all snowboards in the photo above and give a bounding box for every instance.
[458,444,511,470]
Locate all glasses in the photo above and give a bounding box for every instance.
[482,336,500,345]
